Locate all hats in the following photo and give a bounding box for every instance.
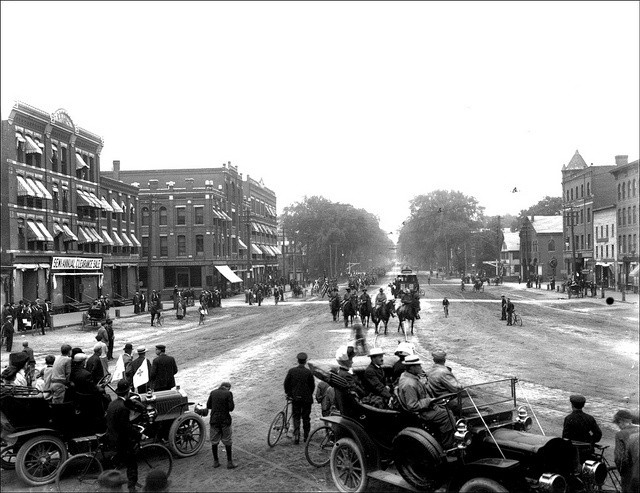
[6,315,13,318]
[362,288,367,292]
[154,301,157,306]
[4,303,10,306]
[570,395,585,405]
[432,349,446,359]
[612,410,632,423]
[501,294,505,297]
[367,348,385,357]
[297,353,307,360]
[336,346,357,361]
[136,292,139,294]
[48,301,51,303]
[394,342,413,355]
[72,353,87,361]
[258,290,261,292]
[10,352,29,363]
[135,345,148,353]
[2,366,17,380]
[444,296,447,298]
[142,470,173,492]
[108,321,112,325]
[123,344,133,350]
[401,355,424,365]
[115,380,130,394]
[345,287,350,290]
[94,345,102,352]
[45,299,49,302]
[97,470,128,487]
[156,345,165,348]
[152,290,156,292]
[379,288,384,291]
[101,321,106,325]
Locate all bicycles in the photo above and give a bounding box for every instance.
[505,310,522,326]
[591,443,622,493]
[306,411,342,467]
[268,394,294,447]
[32,320,46,336]
[154,309,164,329]
[56,424,173,493]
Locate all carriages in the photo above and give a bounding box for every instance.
[82,297,109,330]
[567,285,583,299]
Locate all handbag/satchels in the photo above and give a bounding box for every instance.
[290,386,308,410]
[199,309,208,315]
[23,320,32,327]
[22,317,28,324]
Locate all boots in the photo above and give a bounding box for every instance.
[212,443,220,468]
[304,419,310,442]
[226,445,238,469]
[294,421,300,444]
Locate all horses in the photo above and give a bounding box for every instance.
[395,298,421,336]
[371,299,397,335]
[327,295,341,323]
[341,294,358,328]
[359,298,373,329]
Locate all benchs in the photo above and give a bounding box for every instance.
[309,354,408,435]
[2,365,52,428]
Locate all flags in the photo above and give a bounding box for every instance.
[133,358,150,388]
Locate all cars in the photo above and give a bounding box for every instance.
[307,356,607,493]
[394,274,418,298]
[0,373,209,486]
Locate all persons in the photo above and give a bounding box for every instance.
[336,346,367,398]
[2,352,29,398]
[364,348,392,399]
[460,281,466,293]
[3,315,14,352]
[611,410,640,493]
[86,344,104,377]
[95,321,110,346]
[316,381,335,436]
[90,294,111,318]
[107,380,141,493]
[506,298,513,326]
[284,352,315,445]
[244,283,283,306]
[130,284,222,326]
[93,335,109,376]
[562,395,602,454]
[443,297,449,318]
[132,345,152,394]
[0,297,51,334]
[72,353,93,394]
[107,320,114,360]
[398,354,456,449]
[207,381,238,469]
[391,342,419,382]
[50,344,73,404]
[21,340,35,386]
[121,344,132,386]
[291,260,424,331]
[150,345,177,392]
[36,355,55,400]
[499,296,507,320]
[426,349,473,416]
